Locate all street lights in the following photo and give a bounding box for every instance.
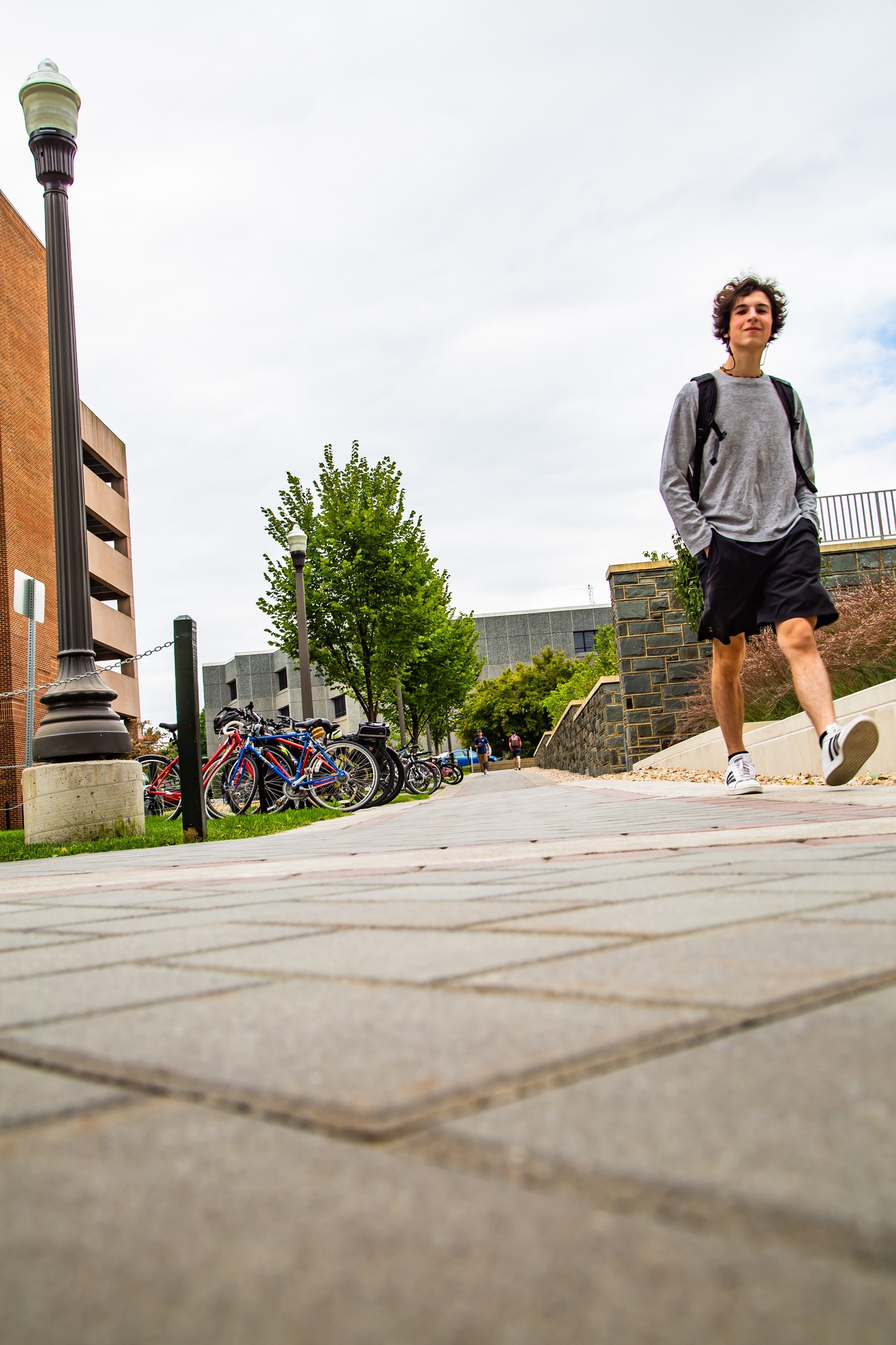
[286,524,314,723]
[16,56,142,847]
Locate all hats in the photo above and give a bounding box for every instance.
[478,730,482,732]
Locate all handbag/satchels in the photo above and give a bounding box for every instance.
[358,722,390,741]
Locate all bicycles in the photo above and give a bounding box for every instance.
[135,701,464,822]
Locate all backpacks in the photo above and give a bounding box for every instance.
[511,735,518,746]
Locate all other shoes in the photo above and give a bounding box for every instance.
[518,768,521,770]
[514,768,518,770]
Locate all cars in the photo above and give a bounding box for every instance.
[433,749,498,771]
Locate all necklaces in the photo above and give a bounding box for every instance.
[720,366,763,378]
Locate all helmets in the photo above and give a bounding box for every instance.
[213,709,241,735]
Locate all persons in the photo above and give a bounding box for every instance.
[474,730,492,776]
[659,278,879,796]
[509,731,522,770]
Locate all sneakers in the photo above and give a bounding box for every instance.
[724,753,762,795]
[822,715,878,786]
[485,769,489,775]
[482,772,484,776]
